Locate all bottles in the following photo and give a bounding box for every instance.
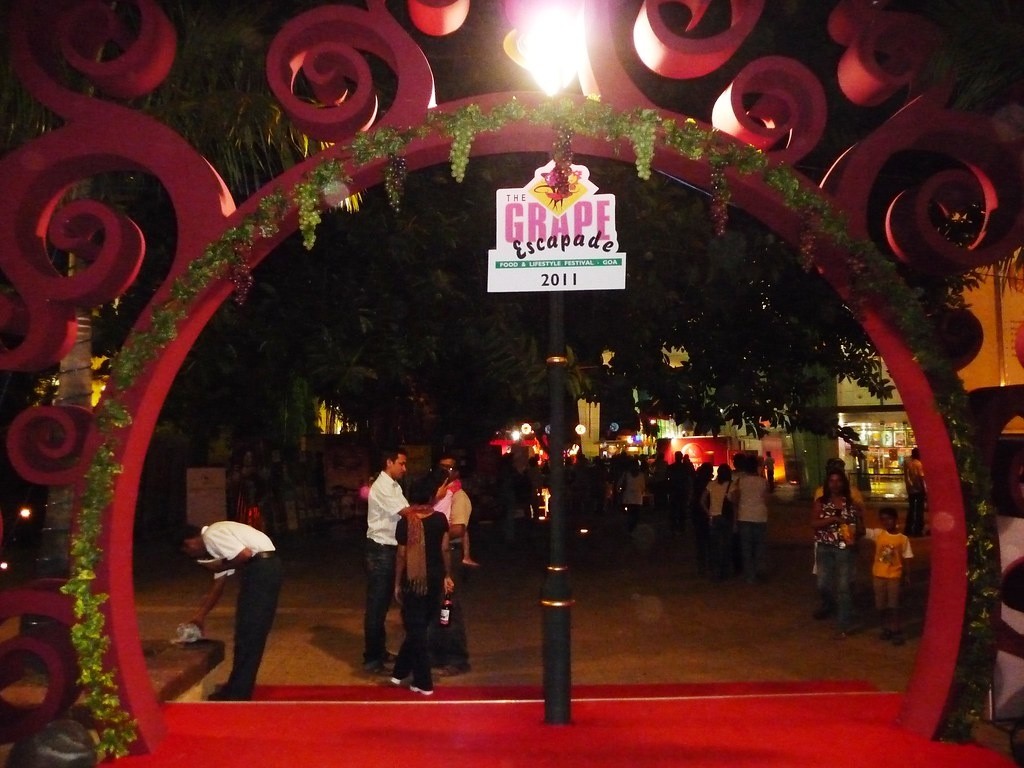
[840,523,853,547]
[439,592,453,627]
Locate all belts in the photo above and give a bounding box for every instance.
[258,550,277,558]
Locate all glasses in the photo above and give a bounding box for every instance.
[439,464,457,471]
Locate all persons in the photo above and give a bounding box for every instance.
[862,507,913,645]
[904,448,926,537]
[362,447,449,676]
[507,453,550,523]
[811,469,864,638]
[813,458,864,620]
[390,478,454,695]
[175,521,284,701]
[565,451,774,586]
[430,454,481,676]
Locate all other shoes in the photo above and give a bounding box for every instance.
[390,676,402,684]
[879,628,893,640]
[385,650,398,661]
[368,664,394,676]
[430,660,468,677]
[410,681,434,695]
[891,628,906,646]
[207,684,252,702]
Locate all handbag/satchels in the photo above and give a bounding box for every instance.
[721,479,735,526]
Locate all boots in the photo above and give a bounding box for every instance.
[814,591,835,620]
